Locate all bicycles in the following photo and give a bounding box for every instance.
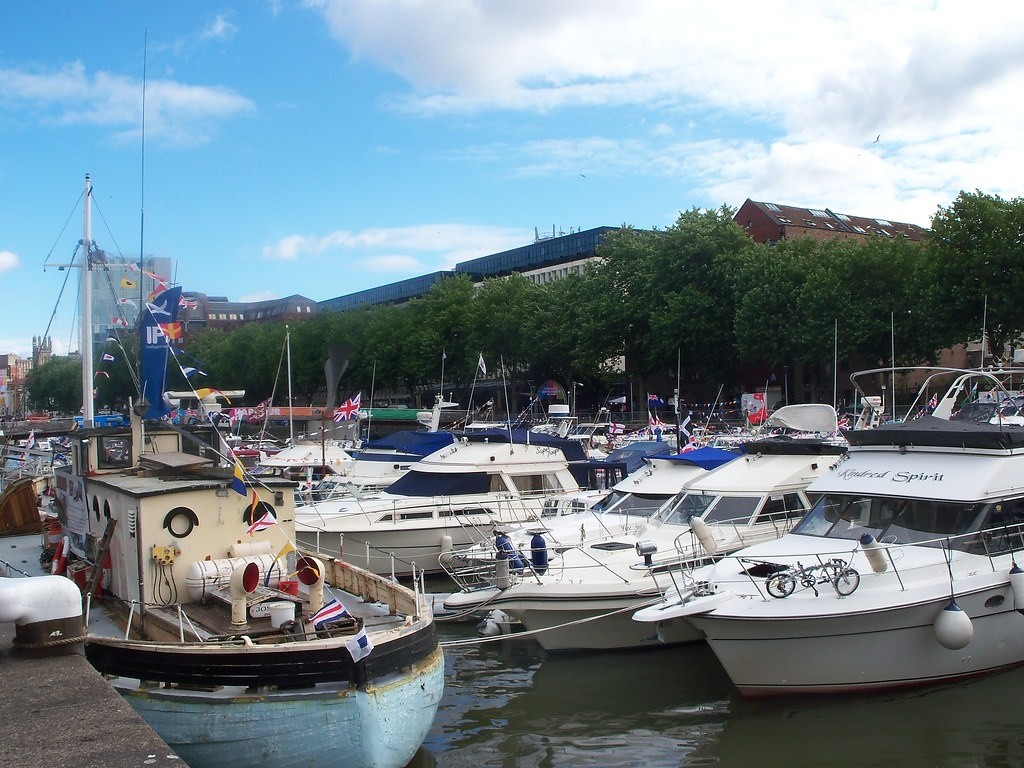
[765,558,860,599]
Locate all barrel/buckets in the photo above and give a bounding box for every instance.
[270,601,296,629]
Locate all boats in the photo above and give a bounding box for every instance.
[218,320,1023,588]
[632,291,1024,698]
[0,173,445,768]
[436,310,896,651]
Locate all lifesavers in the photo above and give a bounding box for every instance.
[51,535,69,575]
[93,537,110,590]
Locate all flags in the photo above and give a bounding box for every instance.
[648,395,756,454]
[478,354,486,375]
[22,260,375,664]
